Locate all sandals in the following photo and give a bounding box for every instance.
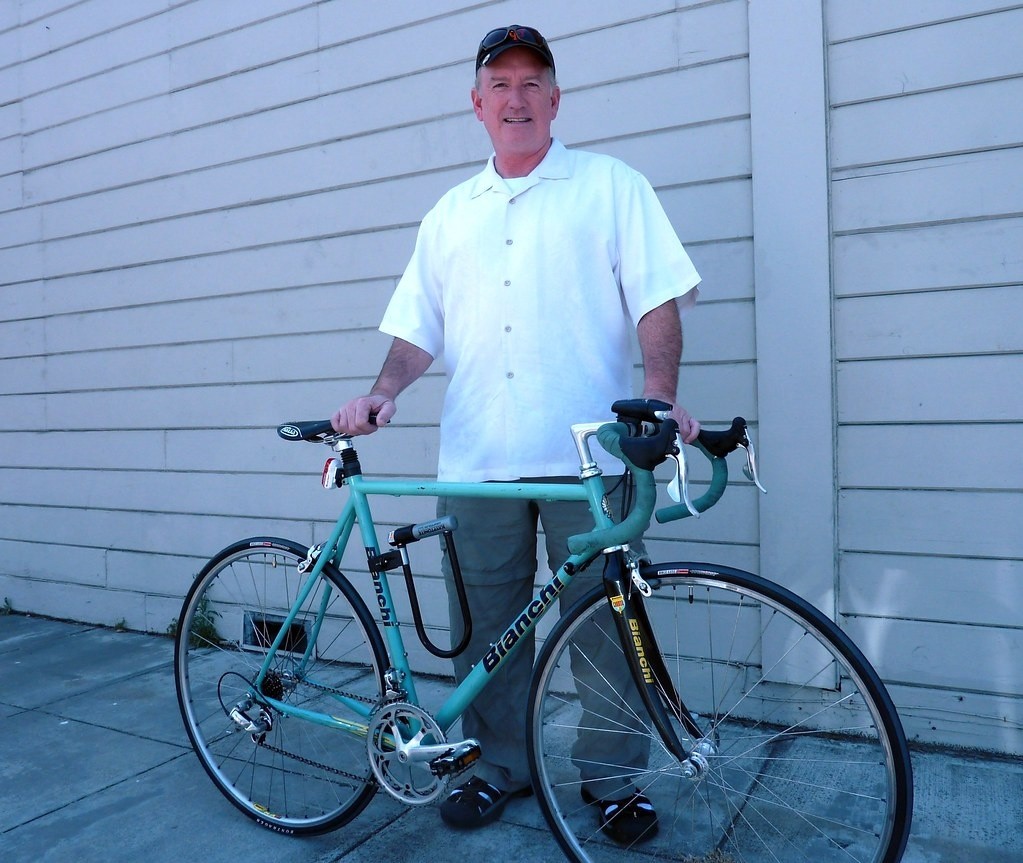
[581,786,658,843]
[441,775,534,829]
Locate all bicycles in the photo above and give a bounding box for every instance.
[173,396,915,863]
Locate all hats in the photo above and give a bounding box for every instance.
[475,24,555,79]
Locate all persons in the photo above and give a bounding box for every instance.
[331,27,704,847]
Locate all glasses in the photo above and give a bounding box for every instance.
[476,26,555,76]
